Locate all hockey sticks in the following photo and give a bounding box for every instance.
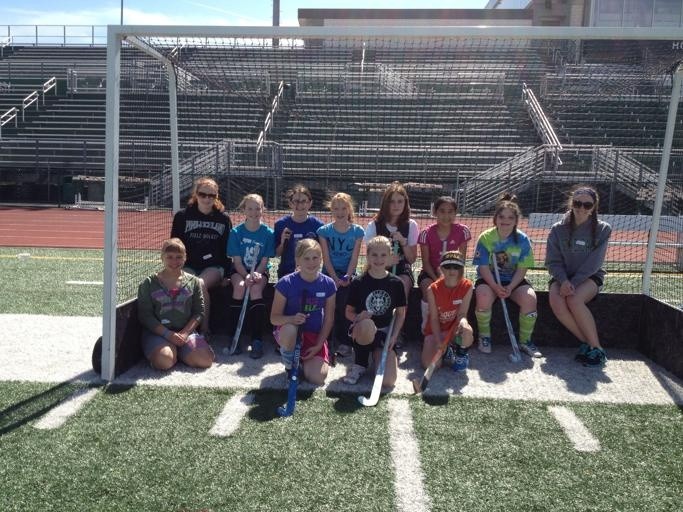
[278,329,303,418]
[359,310,397,405]
[492,254,522,363]
[223,245,259,356]
[414,321,458,397]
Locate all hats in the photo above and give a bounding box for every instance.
[440,250,466,268]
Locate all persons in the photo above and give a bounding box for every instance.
[274,186,327,286]
[346,236,406,387]
[421,250,473,373]
[135,237,214,371]
[171,179,232,342]
[545,188,612,367]
[225,193,274,358]
[473,200,542,358]
[316,193,366,355]
[364,182,419,348]
[418,195,472,334]
[270,238,337,384]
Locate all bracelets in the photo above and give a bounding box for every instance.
[402,239,409,246]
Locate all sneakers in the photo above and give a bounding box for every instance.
[343,364,367,384]
[443,343,454,361]
[201,327,216,345]
[285,368,301,385]
[452,352,471,373]
[517,341,544,358]
[574,342,607,369]
[248,340,265,360]
[227,337,243,355]
[477,335,493,355]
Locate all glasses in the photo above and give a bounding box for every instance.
[196,190,217,200]
[571,199,594,211]
[442,263,463,270]
